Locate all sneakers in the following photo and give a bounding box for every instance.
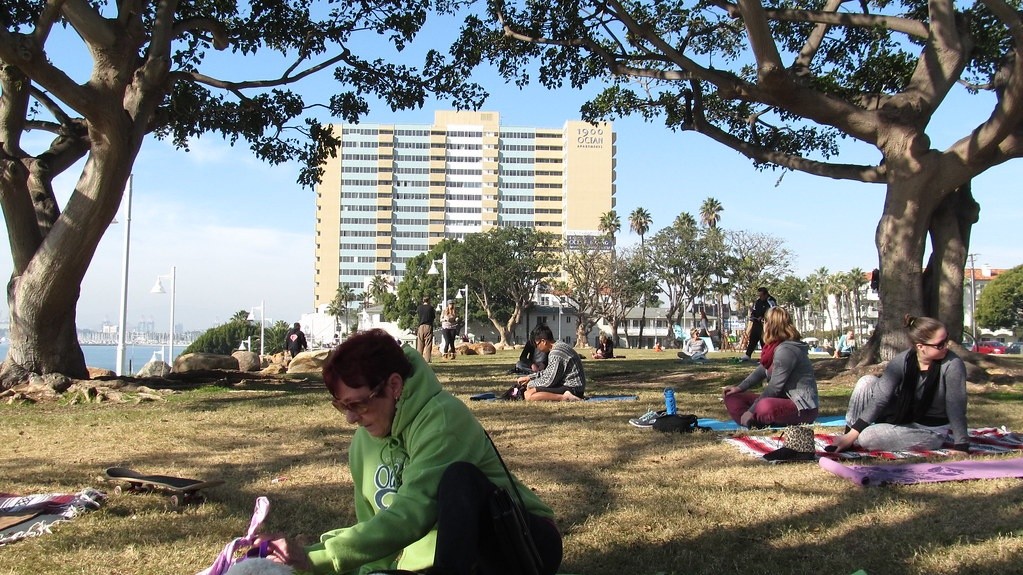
[629,411,667,427]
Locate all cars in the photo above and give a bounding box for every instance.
[971,341,1008,354]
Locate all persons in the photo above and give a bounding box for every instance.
[825,314,969,454]
[724,306,817,428]
[418,295,613,398]
[254,328,562,575]
[657,287,858,359]
[285,322,309,357]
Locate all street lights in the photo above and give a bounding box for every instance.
[427,252,468,346]
[237,336,251,352]
[150,346,165,362]
[151,266,176,368]
[247,299,265,354]
[683,295,690,349]
[112,174,134,377]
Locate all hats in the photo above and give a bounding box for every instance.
[763,428,816,461]
[448,299,454,303]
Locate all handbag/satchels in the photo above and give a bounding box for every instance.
[653,414,699,432]
[502,381,526,401]
[205,495,274,575]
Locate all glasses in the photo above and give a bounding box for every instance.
[330,381,385,415]
[922,332,951,351]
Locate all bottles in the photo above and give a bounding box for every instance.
[664,386,676,415]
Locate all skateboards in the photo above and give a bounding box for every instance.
[105,467,227,508]
